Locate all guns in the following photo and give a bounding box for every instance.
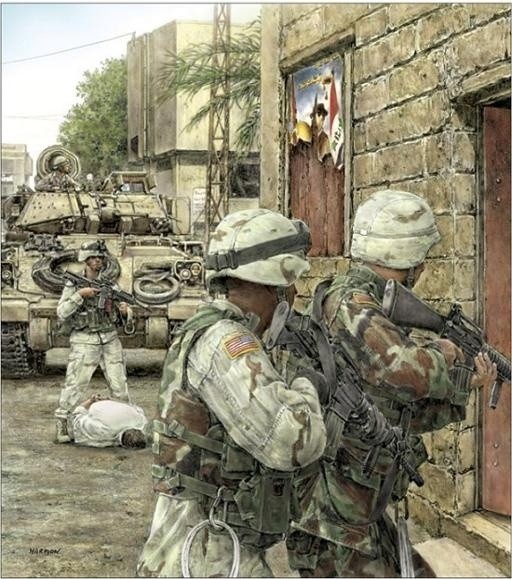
[62,268,153,313]
[383,278,512,410]
[263,301,425,488]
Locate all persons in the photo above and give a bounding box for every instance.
[72,393,147,451]
[309,103,329,158]
[136,208,385,579]
[53,238,133,446]
[286,190,497,578]
[36,156,81,193]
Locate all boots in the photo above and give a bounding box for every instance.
[55,418,72,444]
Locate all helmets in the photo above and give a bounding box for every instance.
[204,208,312,290]
[347,190,441,270]
[77,239,106,262]
